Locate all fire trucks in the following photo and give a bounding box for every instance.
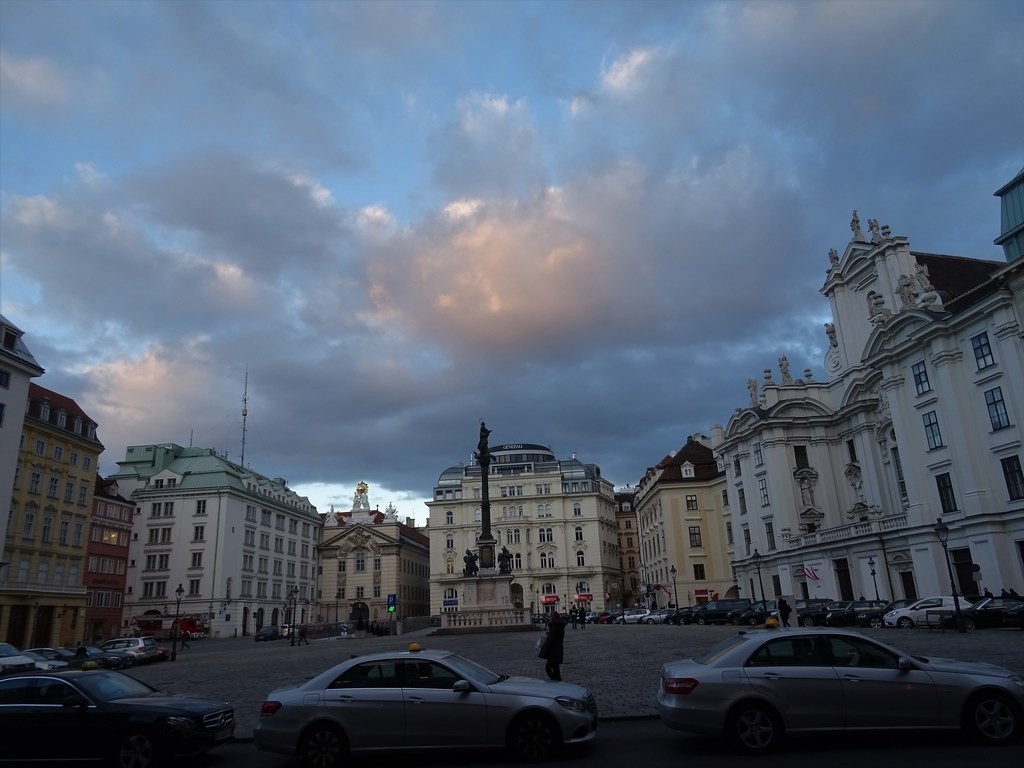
[127,614,205,641]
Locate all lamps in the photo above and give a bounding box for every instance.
[33,602,41,618]
[58,603,68,619]
[219,602,227,615]
[164,607,168,614]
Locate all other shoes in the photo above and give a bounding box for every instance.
[298,644,301,646]
[307,643,310,645]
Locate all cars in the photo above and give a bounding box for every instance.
[254,649,599,768]
[532,604,704,624]
[728,596,1024,630]
[255,626,278,642]
[657,628,1024,755]
[0,668,236,768]
[0,643,131,679]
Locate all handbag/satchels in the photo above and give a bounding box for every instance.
[572,612,576,616]
[535,634,546,651]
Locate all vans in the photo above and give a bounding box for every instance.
[693,598,751,625]
[96,636,161,664]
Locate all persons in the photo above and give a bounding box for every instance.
[778,600,790,627]
[747,379,759,407]
[539,612,568,681]
[569,605,586,629]
[779,356,793,385]
[353,492,370,509]
[180,630,189,651]
[1001,588,1017,595]
[802,480,812,506]
[894,262,933,306]
[298,624,310,646]
[76,644,86,655]
[824,323,838,346]
[651,601,657,609]
[983,587,992,596]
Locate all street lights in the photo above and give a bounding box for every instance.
[933,517,966,633]
[536,588,539,624]
[868,557,880,599]
[670,565,681,625]
[171,584,185,660]
[290,586,299,646]
[618,579,626,625]
[752,549,769,620]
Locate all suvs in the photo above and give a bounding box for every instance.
[279,624,297,639]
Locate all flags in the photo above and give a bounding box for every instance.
[802,567,818,580]
